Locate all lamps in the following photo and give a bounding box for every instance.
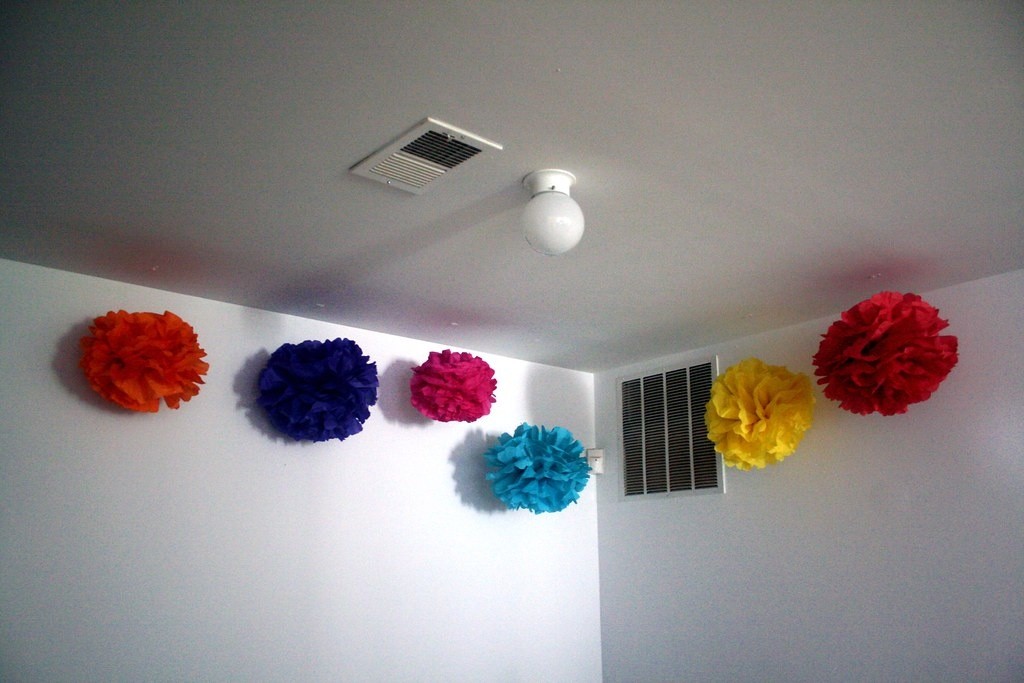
[518,168,586,257]
